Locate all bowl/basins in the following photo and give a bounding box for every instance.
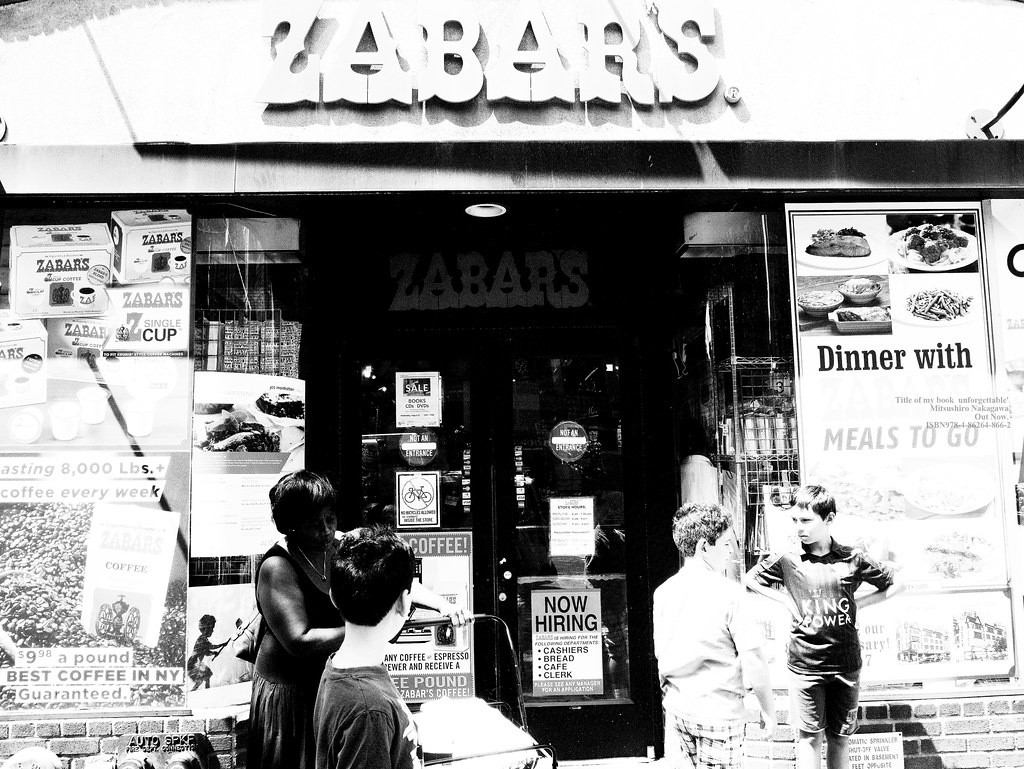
[837,282,882,304]
[796,290,845,318]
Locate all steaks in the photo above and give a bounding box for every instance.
[805,236,871,257]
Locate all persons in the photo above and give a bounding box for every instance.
[312,524,425,769]
[744,482,904,767]
[652,502,779,769]
[290,535,330,582]
[246,469,476,769]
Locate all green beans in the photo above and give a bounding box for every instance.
[907,289,973,321]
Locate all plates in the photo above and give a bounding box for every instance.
[888,306,983,328]
[882,228,978,272]
[796,237,898,271]
[899,488,994,516]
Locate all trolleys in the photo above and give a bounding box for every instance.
[399,615,559,769]
